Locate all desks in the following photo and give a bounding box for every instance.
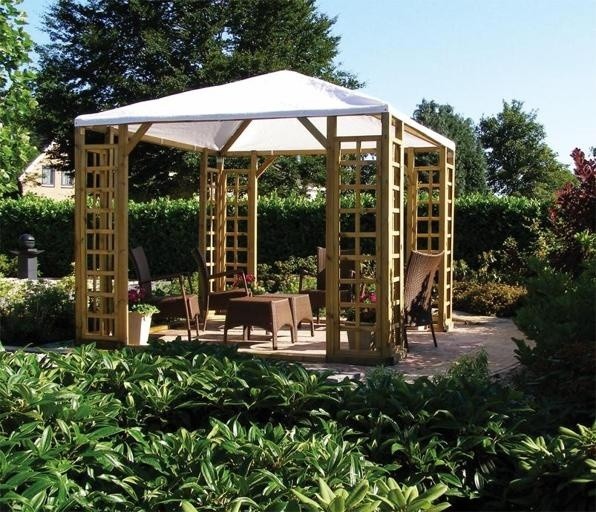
[224,296,295,350]
[263,294,314,341]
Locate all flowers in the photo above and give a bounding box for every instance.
[233,272,265,296]
[342,283,377,322]
[128,286,161,317]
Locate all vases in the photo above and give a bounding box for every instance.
[345,322,377,352]
[128,314,152,345]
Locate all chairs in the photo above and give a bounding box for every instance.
[300,246,363,324]
[395,250,445,352]
[190,248,255,334]
[130,246,200,341]
